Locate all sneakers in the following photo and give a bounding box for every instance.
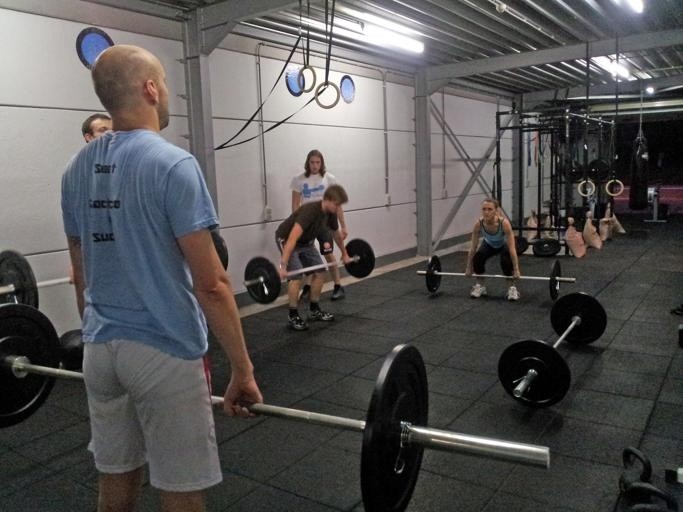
[307,309,333,322]
[299,284,312,302]
[331,284,345,301]
[506,285,520,300]
[469,283,487,298]
[288,314,306,331]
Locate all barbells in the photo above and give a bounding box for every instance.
[0,231,228,310]
[497,292,607,410]
[513,234,568,255]
[241,239,375,305]
[417,256,574,300]
[0,302,550,512]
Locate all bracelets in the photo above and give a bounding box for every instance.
[466,263,472,268]
[279,261,288,271]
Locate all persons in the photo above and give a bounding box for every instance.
[59,44,262,510]
[81,113,113,144]
[462,197,521,301]
[274,184,351,331]
[289,149,349,301]
